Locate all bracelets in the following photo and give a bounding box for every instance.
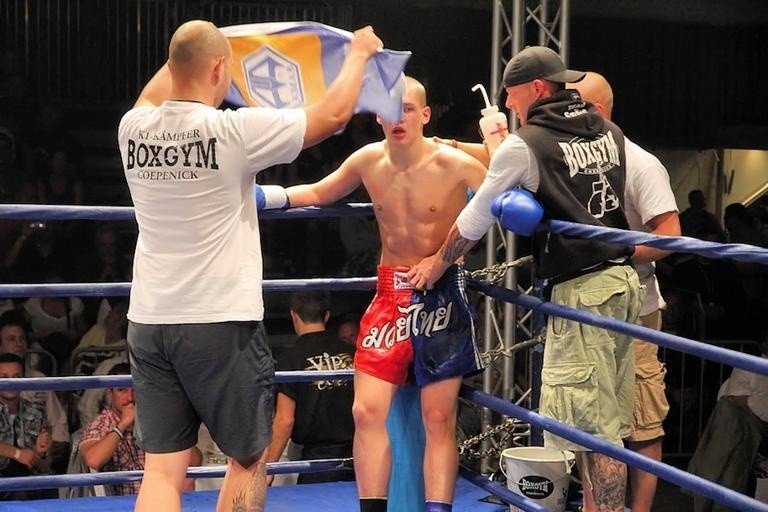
[450,139,457,148]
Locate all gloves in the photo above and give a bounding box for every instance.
[490,186,546,240]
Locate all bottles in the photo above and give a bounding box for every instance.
[480,104,508,162]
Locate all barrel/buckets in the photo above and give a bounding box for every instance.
[499,447,576,512]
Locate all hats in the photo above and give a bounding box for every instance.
[500,44,587,89]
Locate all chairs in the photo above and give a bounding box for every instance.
[24,345,128,429]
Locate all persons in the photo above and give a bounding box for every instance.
[406,45,647,511]
[117,18,383,511]
[6,127,768,511]
[427,70,689,511]
[256,75,544,511]
